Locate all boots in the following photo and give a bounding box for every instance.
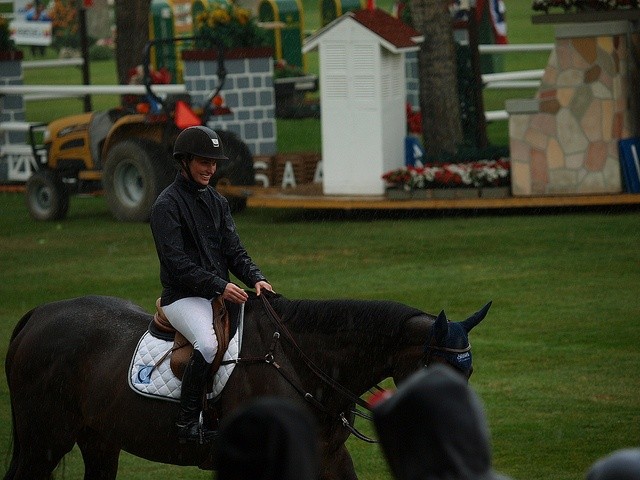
[175,349,220,439]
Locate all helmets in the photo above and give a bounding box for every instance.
[173,125,229,160]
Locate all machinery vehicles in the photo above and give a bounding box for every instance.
[25,37,255,224]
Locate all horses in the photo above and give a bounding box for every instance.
[2,295,492,480]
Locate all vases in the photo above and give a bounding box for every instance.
[409,189,431,199]
[431,190,455,200]
[481,188,509,200]
[385,188,411,200]
[456,188,481,200]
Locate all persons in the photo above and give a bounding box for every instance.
[148,125,275,445]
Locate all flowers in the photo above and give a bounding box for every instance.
[383,167,413,187]
[413,162,435,187]
[480,157,510,188]
[456,161,482,188]
[435,162,456,188]
